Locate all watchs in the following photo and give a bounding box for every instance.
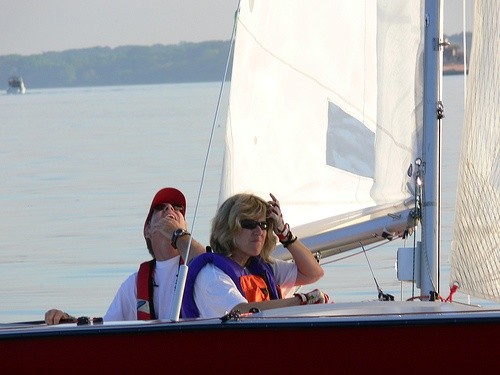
[171,227,191,250]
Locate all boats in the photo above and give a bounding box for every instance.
[1,0,500,374]
[6,75,27,95]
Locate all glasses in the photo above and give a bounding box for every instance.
[153,201,184,211]
[240,218,269,231]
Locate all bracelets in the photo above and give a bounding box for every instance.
[277,233,298,248]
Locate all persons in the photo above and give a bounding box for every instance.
[45,188,213,325]
[181,192,329,319]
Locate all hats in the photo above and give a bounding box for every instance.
[144,187,187,241]
